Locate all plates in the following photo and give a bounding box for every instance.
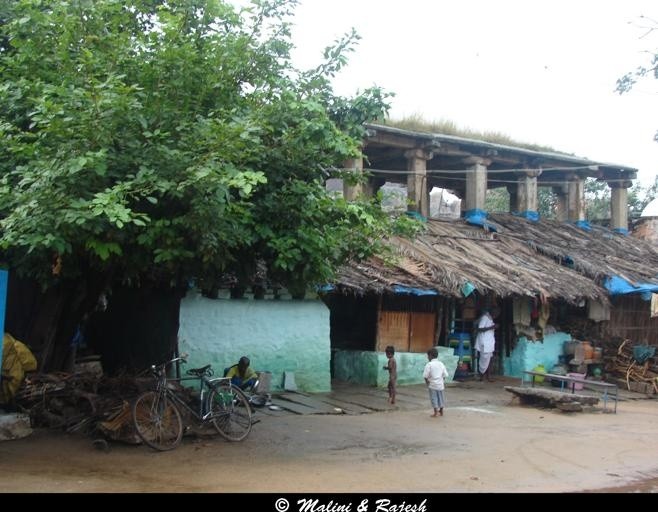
[269,406,285,411]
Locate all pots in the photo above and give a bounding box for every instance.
[236,392,271,407]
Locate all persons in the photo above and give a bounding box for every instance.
[474,305,501,382]
[383,346,397,404]
[423,348,449,418]
[223,356,258,391]
[472,310,482,337]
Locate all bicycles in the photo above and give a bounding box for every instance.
[132,357,252,451]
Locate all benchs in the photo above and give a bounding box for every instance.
[520,370,619,415]
